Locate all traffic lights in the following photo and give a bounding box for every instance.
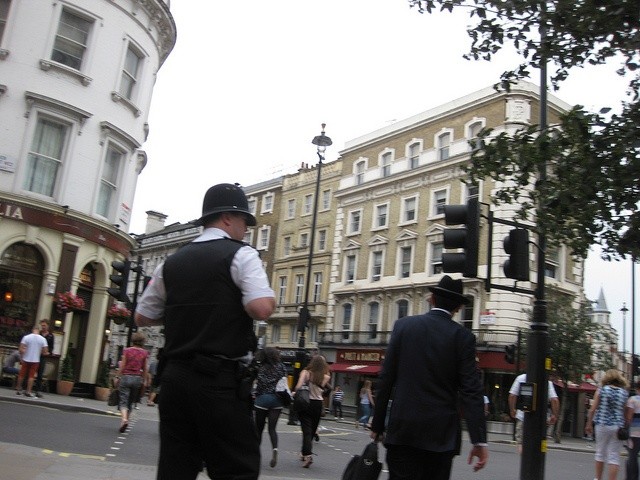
[442,198,479,277]
[145,277,151,289]
[504,229,528,280]
[108,259,130,302]
[505,346,514,364]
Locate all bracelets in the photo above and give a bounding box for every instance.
[115,373,122,380]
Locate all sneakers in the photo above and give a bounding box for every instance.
[270,448,278,467]
[302,456,313,468]
[119,419,128,433]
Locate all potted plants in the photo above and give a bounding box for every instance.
[56,354,75,395]
[95,361,111,401]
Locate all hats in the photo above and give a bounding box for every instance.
[191,182,258,227]
[427,274,473,305]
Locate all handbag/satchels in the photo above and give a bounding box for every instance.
[618,426,629,441]
[107,379,121,406]
[293,370,312,413]
[341,430,383,480]
[275,363,293,408]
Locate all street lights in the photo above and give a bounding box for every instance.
[620,302,629,351]
[288,123,332,425]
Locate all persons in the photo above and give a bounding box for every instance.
[113,331,149,432]
[16,319,54,395]
[355,380,375,429]
[368,274,489,480]
[147,354,158,407]
[15,324,49,397]
[134,183,279,479]
[118,354,124,369]
[483,395,490,436]
[508,372,560,453]
[332,385,345,420]
[132,364,146,409]
[625,380,639,480]
[2,344,20,391]
[584,369,630,480]
[294,354,331,469]
[255,346,287,467]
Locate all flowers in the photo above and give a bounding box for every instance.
[108,305,132,317]
[52,291,85,309]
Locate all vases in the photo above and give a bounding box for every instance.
[64,307,73,312]
[114,317,123,325]
